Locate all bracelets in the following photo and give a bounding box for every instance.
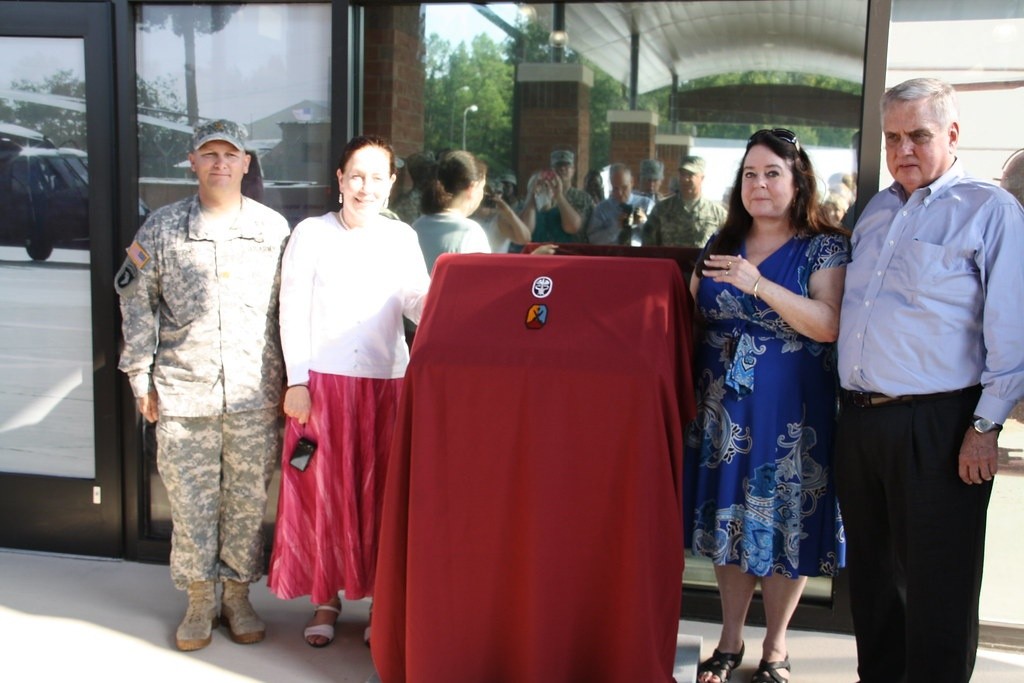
[754,276,763,301]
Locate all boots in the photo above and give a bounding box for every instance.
[222,579,266,643]
[176,580,221,649]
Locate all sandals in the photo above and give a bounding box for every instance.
[363,605,372,648]
[302,600,342,647]
[699,640,746,682]
[749,651,791,683]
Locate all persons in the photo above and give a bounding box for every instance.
[241,150,264,202]
[837,77,1024,683]
[397,150,856,272]
[689,129,849,683]
[116,121,290,650]
[266,137,431,647]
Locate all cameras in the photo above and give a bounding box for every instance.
[624,210,639,228]
[290,437,315,471]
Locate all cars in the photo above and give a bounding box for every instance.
[0,121,152,261]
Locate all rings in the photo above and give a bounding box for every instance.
[727,261,732,266]
[726,270,729,275]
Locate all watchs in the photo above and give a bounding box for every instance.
[969,417,1004,433]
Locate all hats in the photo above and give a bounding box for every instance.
[678,157,706,175]
[499,172,518,184]
[189,118,248,153]
[550,151,574,164]
[640,160,665,181]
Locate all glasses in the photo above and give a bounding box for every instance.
[748,128,802,152]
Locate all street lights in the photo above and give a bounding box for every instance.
[450,86,469,149]
[462,105,478,151]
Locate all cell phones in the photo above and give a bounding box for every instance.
[539,169,554,184]
[480,199,496,209]
